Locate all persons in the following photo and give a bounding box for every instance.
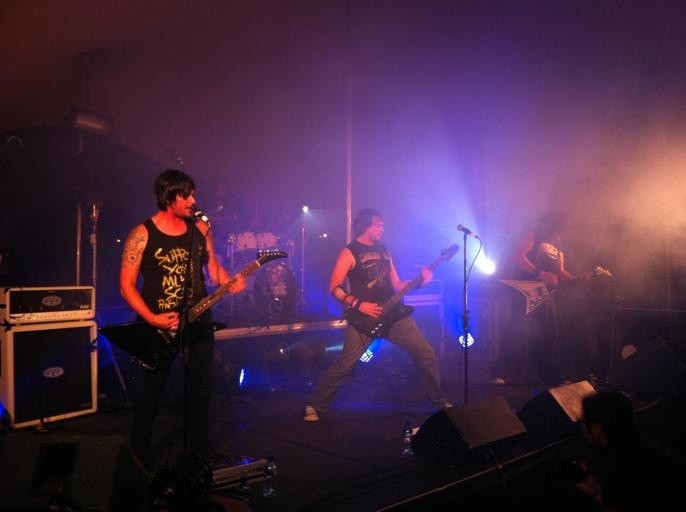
[300,204,455,422]
[109,166,250,512]
[489,208,593,388]
[573,389,686,512]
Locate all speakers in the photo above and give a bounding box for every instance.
[596,335,677,399]
[512,379,598,444]
[410,395,527,476]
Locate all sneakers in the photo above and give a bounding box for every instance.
[302,404,319,422]
[488,376,505,384]
[128,441,160,477]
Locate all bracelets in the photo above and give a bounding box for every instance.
[349,297,362,310]
[590,487,601,497]
[532,266,541,279]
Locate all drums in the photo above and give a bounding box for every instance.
[226,232,258,258]
[231,259,297,317]
[257,232,280,254]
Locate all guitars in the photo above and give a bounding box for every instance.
[98,249,288,371]
[498,265,613,314]
[344,245,458,337]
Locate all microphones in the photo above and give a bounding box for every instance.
[191,206,212,225]
[456,224,477,239]
